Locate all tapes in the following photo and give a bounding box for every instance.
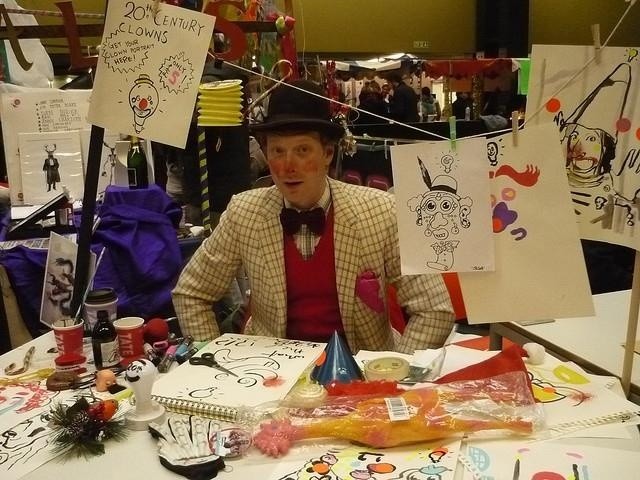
[365,357,410,382]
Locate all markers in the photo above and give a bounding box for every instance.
[143,332,199,373]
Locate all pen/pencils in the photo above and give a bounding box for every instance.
[2,369,56,386]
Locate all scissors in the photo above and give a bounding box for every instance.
[189,353,239,378]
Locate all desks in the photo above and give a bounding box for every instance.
[0,330,640,480]
[0,232,205,354]
[490,289,640,405]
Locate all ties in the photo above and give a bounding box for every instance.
[300,223,315,260]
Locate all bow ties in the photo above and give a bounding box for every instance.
[280,207,326,234]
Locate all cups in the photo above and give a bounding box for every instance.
[52,287,145,363]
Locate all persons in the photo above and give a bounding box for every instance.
[171,126,456,355]
[453,92,473,119]
[349,72,418,124]
[422,86,441,121]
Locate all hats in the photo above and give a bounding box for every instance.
[249,80,344,138]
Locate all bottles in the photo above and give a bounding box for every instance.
[464,104,471,121]
[127,135,148,190]
[92,311,120,371]
